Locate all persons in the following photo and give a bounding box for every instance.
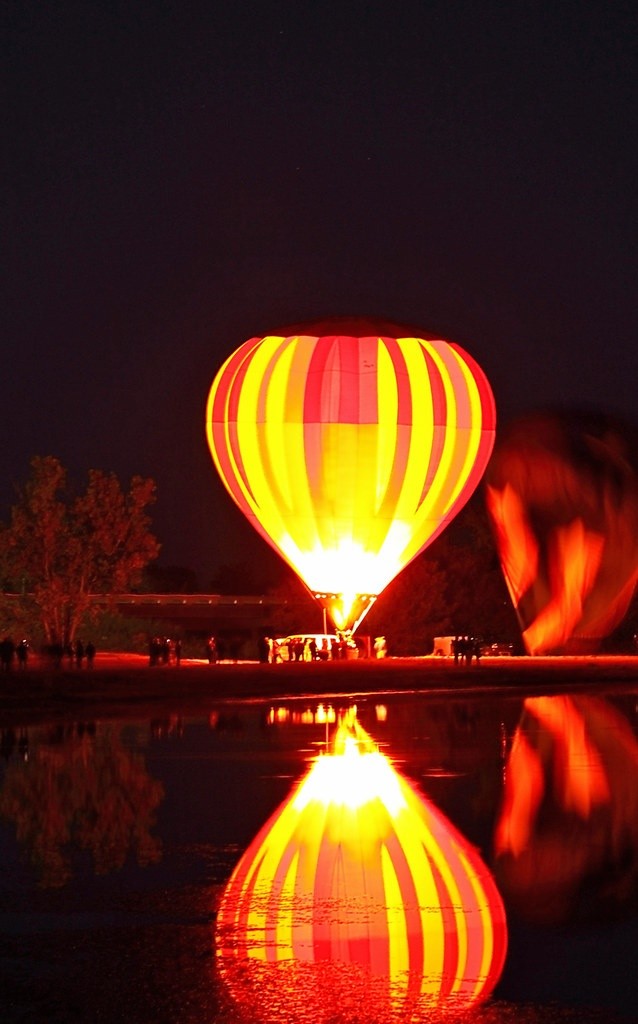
[0,637,27,673]
[53,640,95,669]
[258,631,351,661]
[208,636,216,651]
[149,636,170,667]
[175,640,181,666]
[453,636,482,666]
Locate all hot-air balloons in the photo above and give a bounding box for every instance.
[214,699,511,1024]
[206,318,498,653]
